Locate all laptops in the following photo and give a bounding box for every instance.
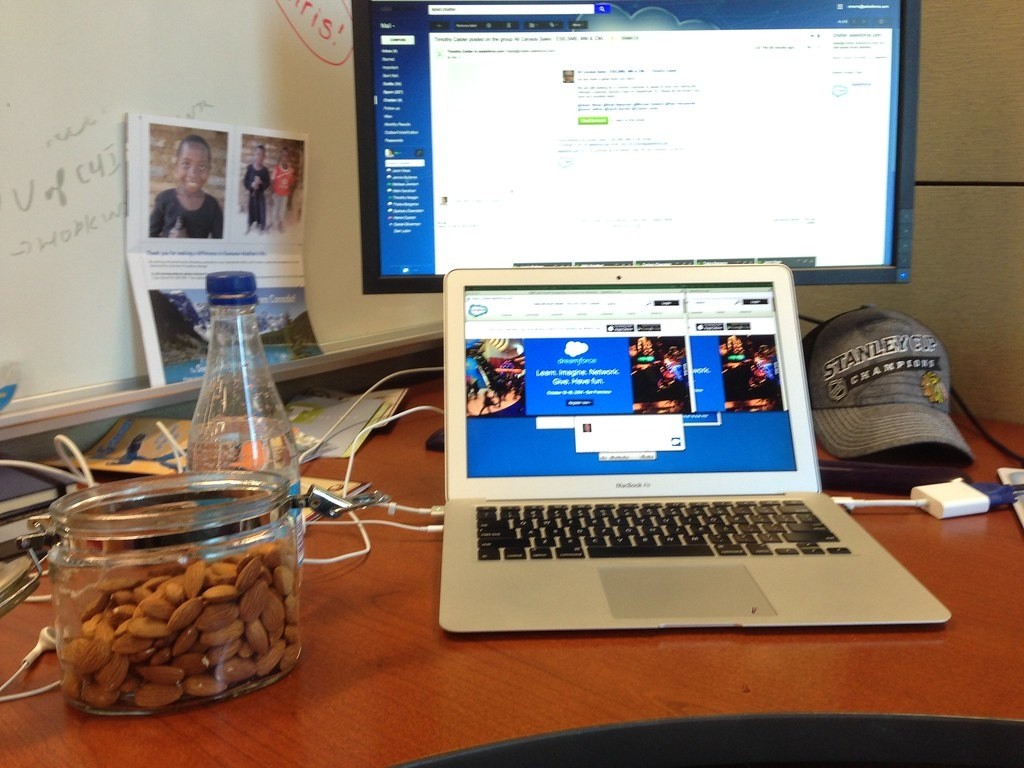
[438,264,952,635]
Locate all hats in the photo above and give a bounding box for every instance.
[802,303,974,463]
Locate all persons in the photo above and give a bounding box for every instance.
[242,144,296,236]
[148,135,224,240]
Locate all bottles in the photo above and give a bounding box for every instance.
[48,470,304,716]
[185,270,303,589]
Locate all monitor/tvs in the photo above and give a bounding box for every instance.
[351,0,922,452]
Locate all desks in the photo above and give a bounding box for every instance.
[0,374,1024,767]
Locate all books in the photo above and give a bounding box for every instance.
[68,416,196,477]
[0,455,77,559]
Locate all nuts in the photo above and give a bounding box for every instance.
[59,537,303,707]
